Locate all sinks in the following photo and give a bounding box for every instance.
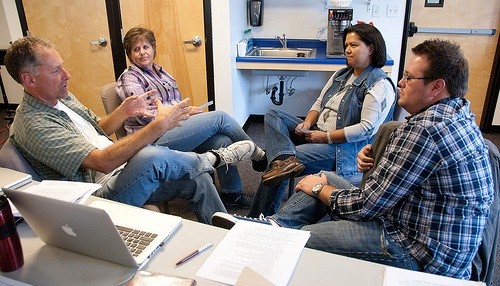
[246,46,318,60]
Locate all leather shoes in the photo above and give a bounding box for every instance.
[262,155,304,183]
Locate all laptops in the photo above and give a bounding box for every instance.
[1,188,183,269]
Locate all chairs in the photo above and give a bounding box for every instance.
[1,137,170,213]
[469,137,500,285]
[286,87,403,199]
[102,81,216,187]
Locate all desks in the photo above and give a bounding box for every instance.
[0,167,486,285]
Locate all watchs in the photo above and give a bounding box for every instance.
[312,183,329,198]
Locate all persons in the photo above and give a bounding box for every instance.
[209,38,495,280]
[114,27,268,208]
[5,36,255,225]
[233,23,397,217]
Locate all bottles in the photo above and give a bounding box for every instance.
[243,28,254,54]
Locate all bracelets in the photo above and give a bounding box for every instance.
[327,129,332,145]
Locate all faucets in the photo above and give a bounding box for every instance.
[274,32,289,49]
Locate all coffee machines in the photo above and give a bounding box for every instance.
[326,0,354,59]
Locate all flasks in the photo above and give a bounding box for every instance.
[0,195,24,273]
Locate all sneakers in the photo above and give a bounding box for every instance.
[213,212,281,231]
[251,149,269,172]
[221,192,253,210]
[208,140,254,168]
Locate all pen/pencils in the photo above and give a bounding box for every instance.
[175,242,213,266]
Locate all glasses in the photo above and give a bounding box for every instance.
[403,71,432,82]
